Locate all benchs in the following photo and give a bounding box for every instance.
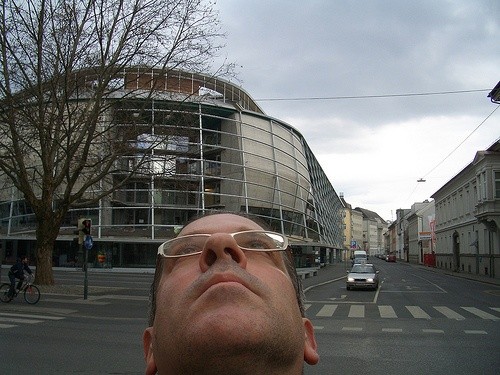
[308,270,318,278]
[296,271,310,280]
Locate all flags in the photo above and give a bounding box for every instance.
[200,86,223,97]
[108,79,124,89]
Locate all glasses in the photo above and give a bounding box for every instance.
[149,229,302,312]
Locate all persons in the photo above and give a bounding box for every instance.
[142,210,320,375]
[106,250,112,268]
[7,256,35,300]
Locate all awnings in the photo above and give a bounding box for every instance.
[470,239,479,248]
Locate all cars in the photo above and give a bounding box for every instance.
[345,262,380,291]
[350,250,397,267]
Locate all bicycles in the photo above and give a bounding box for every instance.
[0,274,40,305]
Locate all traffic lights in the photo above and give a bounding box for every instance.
[72,219,83,245]
[81,219,91,236]
